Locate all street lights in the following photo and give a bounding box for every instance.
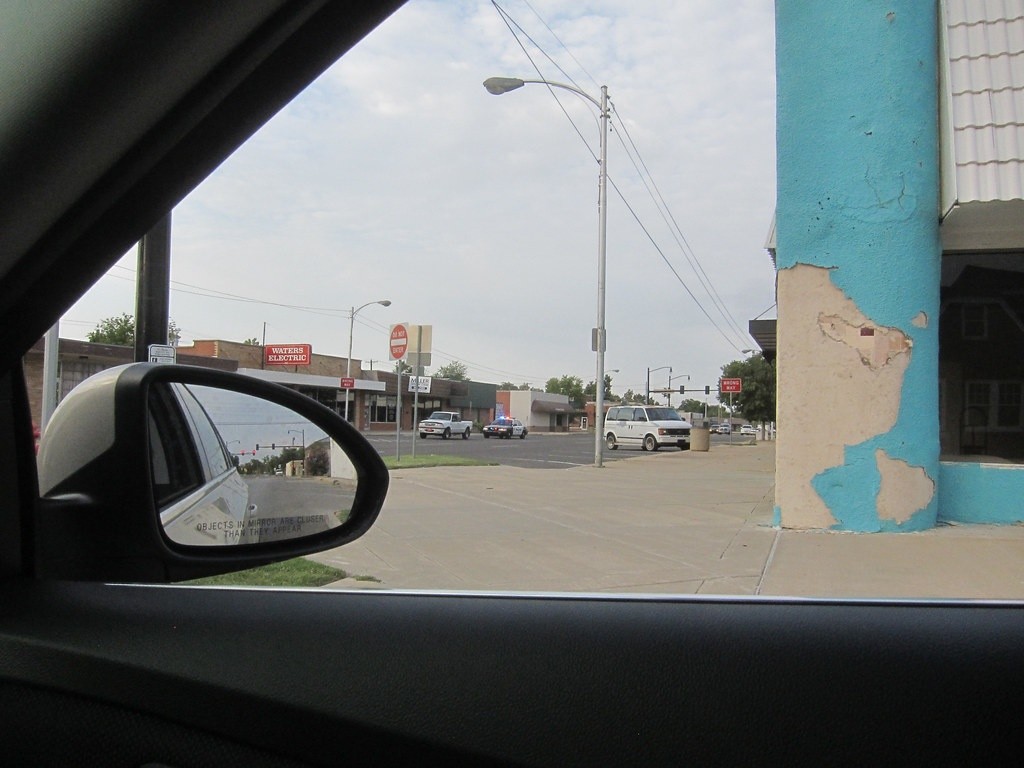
[667,374,689,406]
[225,440,240,451]
[647,367,672,406]
[344,299,392,422]
[288,429,305,476]
[483,74,611,467]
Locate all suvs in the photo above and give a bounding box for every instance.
[602,406,691,451]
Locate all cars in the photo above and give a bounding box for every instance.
[718,423,731,435]
[482,416,529,439]
[709,424,719,434]
[740,424,760,435]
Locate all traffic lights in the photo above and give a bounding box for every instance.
[253,450,256,456]
[241,451,244,456]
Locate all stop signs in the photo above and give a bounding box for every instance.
[389,322,411,361]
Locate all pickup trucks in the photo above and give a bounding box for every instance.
[418,411,474,440]
